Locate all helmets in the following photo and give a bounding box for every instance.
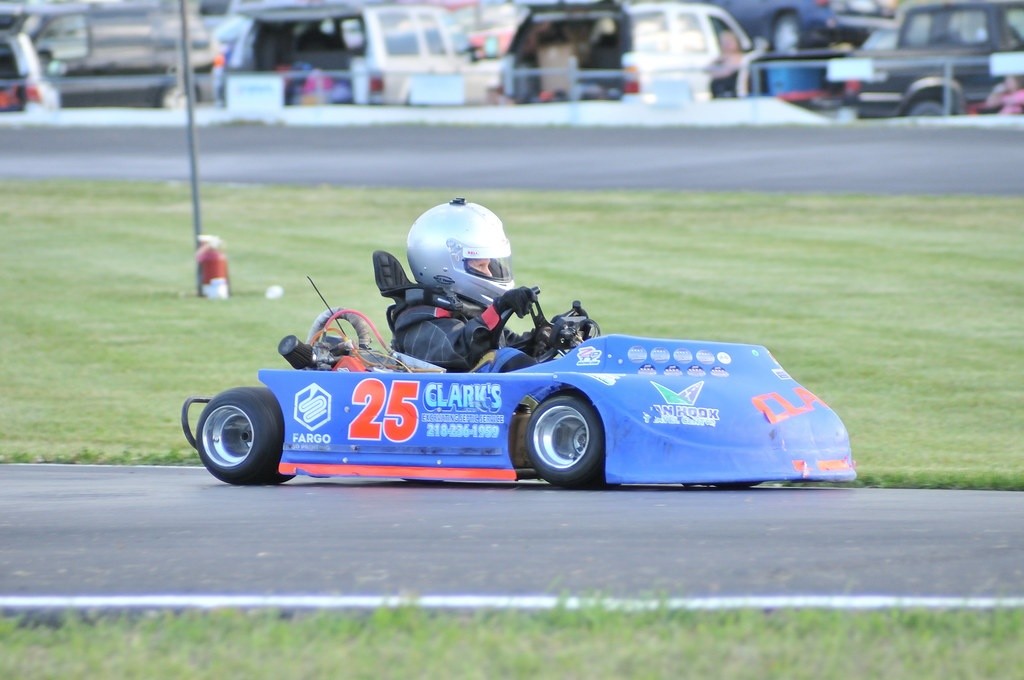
[407,197,516,309]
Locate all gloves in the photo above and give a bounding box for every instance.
[494,286,538,318]
[580,324,592,342]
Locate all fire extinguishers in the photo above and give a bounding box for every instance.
[193,235,232,300]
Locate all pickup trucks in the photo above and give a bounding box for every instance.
[751,2,1023,118]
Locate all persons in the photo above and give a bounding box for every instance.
[0,42,59,114]
[986,76,1024,115]
[480,31,599,109]
[710,32,740,98]
[394,198,600,372]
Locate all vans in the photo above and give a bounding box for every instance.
[498,0,761,106]
[0,0,202,111]
[219,1,462,108]
